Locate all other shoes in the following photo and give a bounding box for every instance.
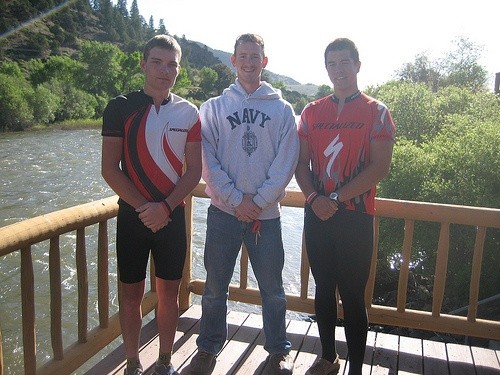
[304,354,340,375]
[143,362,174,375]
[124,365,143,375]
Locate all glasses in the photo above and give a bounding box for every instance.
[235,33,264,46]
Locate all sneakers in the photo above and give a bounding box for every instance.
[261,353,294,375]
[189,350,216,375]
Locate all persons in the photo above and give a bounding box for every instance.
[295,38,395,375]
[101,35,203,375]
[190,32,300,375]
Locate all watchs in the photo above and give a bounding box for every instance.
[329,192,342,205]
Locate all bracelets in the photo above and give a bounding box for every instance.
[162,198,173,214]
[305,192,319,205]
